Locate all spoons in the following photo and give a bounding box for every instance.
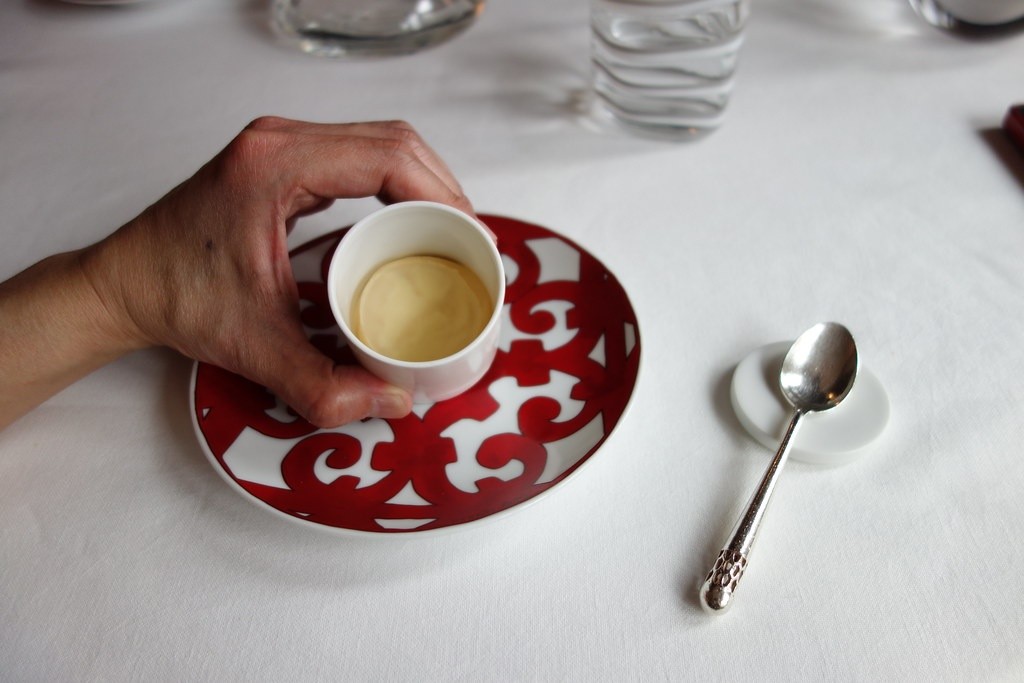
[699,322,859,614]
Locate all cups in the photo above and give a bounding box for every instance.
[327,201,506,404]
[589,0,750,141]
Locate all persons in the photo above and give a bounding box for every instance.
[0,115,497,429]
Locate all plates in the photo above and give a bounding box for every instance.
[187,210,645,538]
